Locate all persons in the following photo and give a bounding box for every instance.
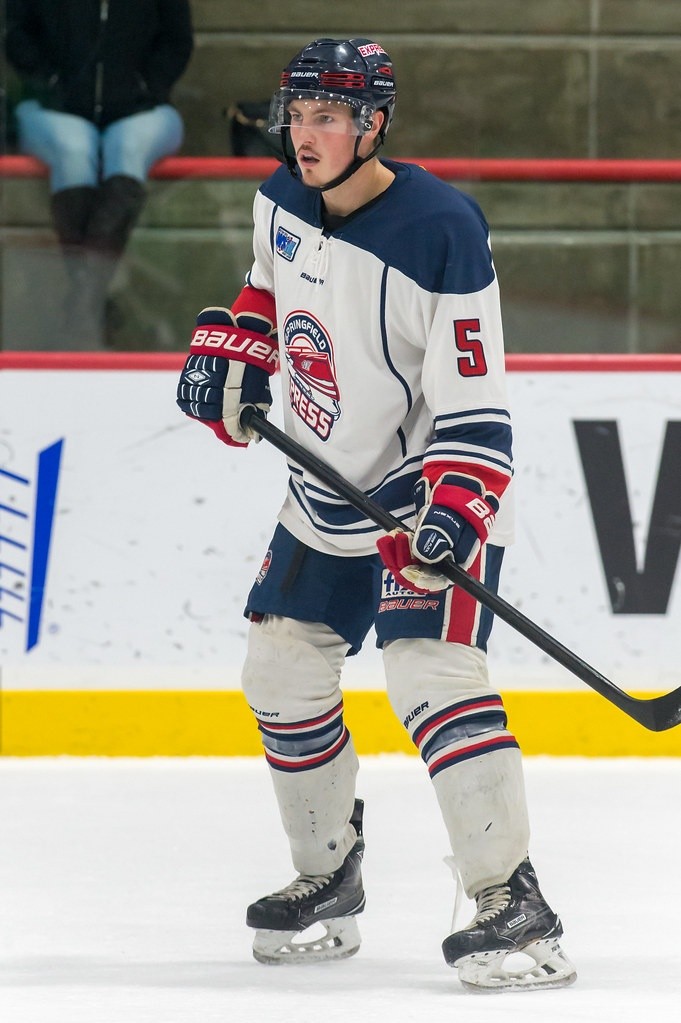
[0,0,194,352]
[177,37,563,970]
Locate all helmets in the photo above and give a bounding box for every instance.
[276,38,396,145]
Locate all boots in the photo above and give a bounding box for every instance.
[48,174,145,349]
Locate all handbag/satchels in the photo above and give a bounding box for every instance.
[226,100,281,156]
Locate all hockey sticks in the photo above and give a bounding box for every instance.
[241,406,681,734]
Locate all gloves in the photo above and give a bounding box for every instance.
[376,471,500,594]
[176,306,280,448]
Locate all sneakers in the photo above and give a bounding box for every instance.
[442,850,577,996]
[246,799,366,965]
